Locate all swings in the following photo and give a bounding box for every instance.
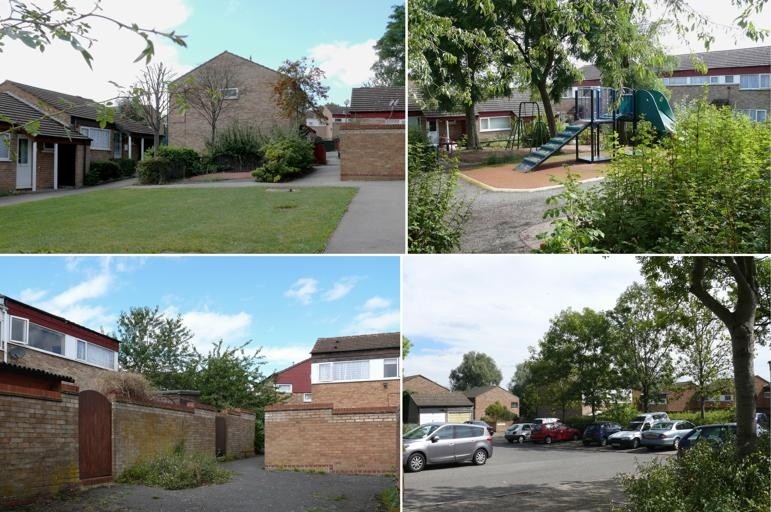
[523,103,535,141]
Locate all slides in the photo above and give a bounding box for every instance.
[635,89,687,141]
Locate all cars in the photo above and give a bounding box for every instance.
[582,412,769,460]
[403,420,494,472]
[504,417,579,444]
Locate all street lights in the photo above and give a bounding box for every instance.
[343,98,350,124]
